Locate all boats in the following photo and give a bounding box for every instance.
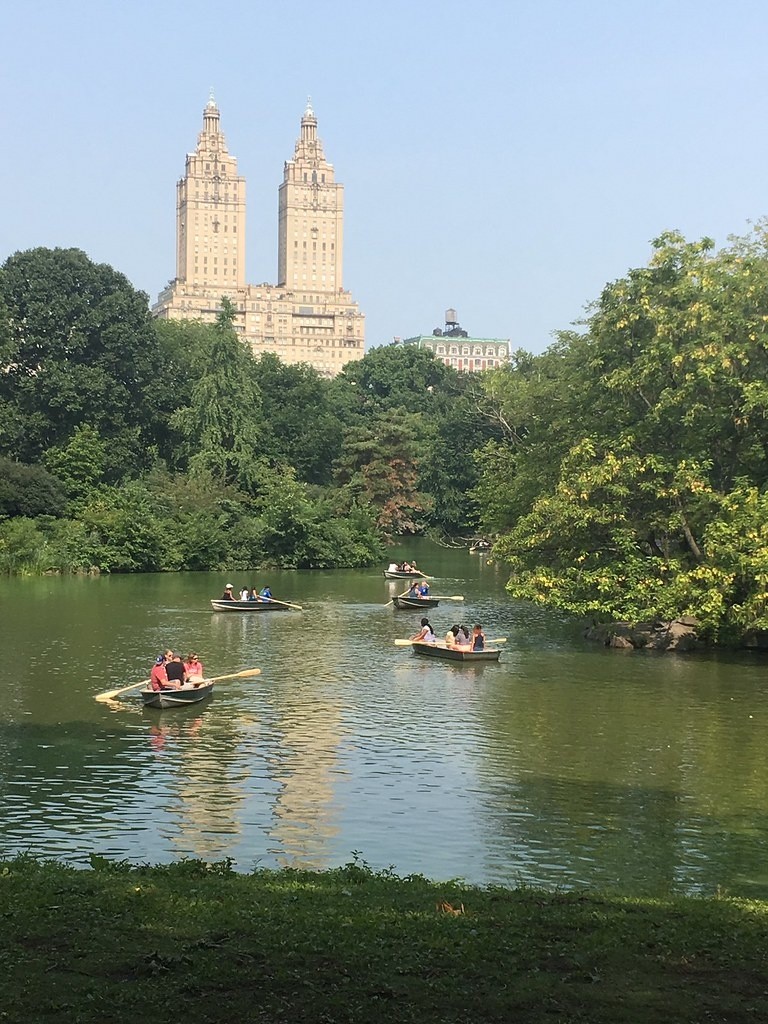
[412,639,503,661]
[210,599,291,612]
[139,678,215,709]
[383,569,424,579]
[392,596,440,609]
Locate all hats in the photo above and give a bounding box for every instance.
[225,583,234,589]
[155,654,165,665]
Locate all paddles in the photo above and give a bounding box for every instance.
[470,637,507,644]
[395,639,452,646]
[421,596,464,600]
[257,595,302,610]
[383,588,411,606]
[193,669,260,684]
[96,678,152,699]
[420,573,433,579]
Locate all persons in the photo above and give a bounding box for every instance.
[388,560,413,572]
[261,587,272,601]
[240,586,249,601]
[163,649,189,685]
[472,624,485,651]
[419,582,429,595]
[221,583,236,601]
[184,653,202,688]
[413,618,436,642]
[249,587,259,600]
[151,653,181,690]
[446,625,459,647]
[411,583,422,597]
[456,625,471,645]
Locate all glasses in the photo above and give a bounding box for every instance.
[167,654,173,657]
[192,657,199,660]
